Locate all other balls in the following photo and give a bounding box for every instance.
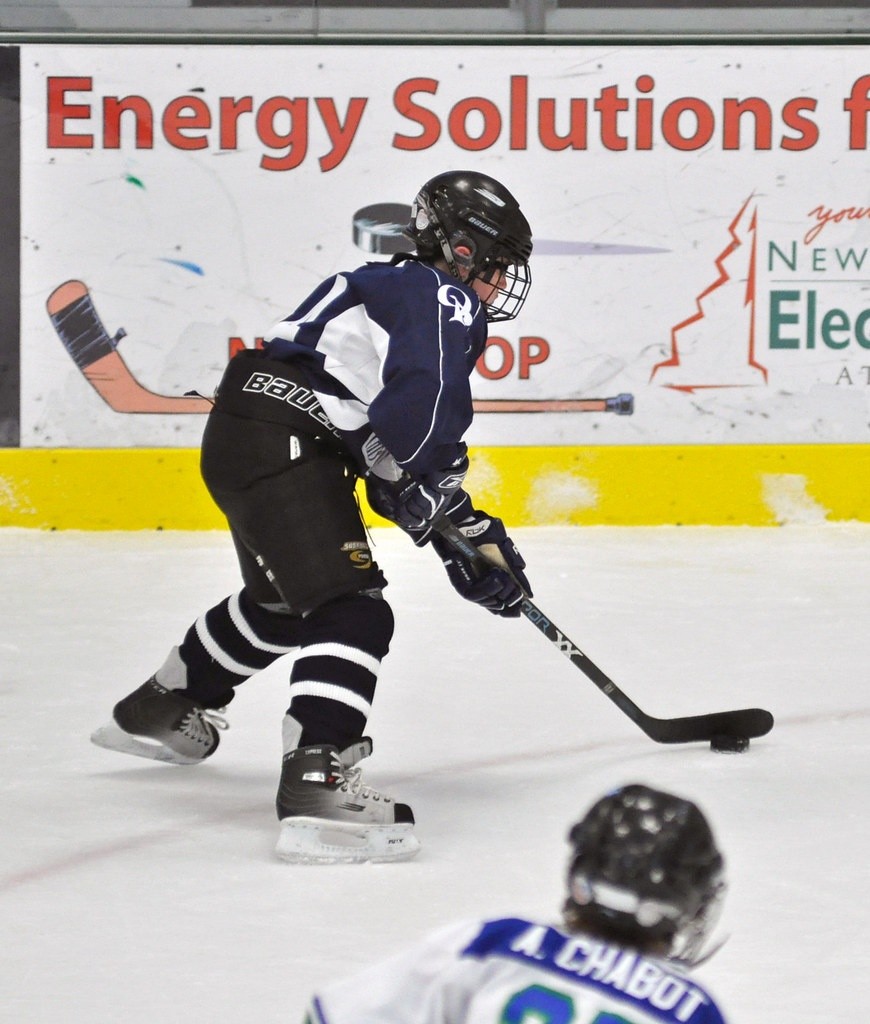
[709,736,748,753]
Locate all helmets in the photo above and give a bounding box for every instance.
[560,784,730,968]
[403,170,533,324]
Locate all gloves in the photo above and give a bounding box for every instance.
[366,437,469,531]
[431,492,532,618]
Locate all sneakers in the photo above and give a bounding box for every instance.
[276,736,419,863]
[89,644,235,764]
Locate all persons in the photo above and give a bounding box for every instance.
[295,785,730,1024]
[84,162,535,865]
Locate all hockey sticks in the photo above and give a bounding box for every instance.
[432,514,773,745]
